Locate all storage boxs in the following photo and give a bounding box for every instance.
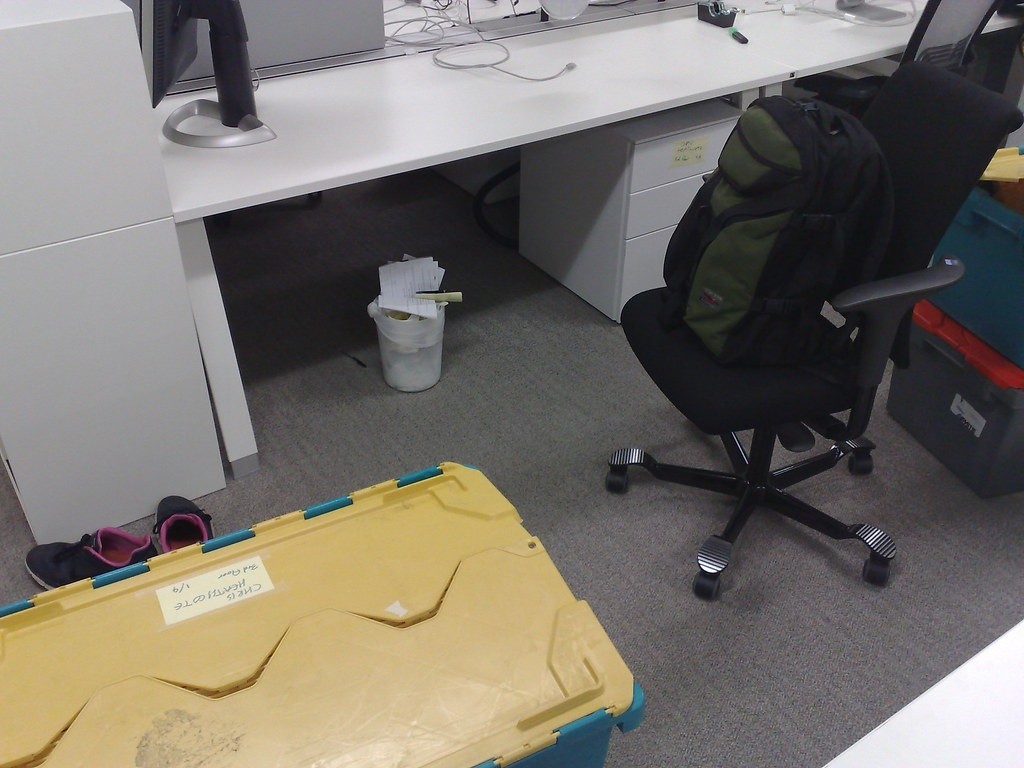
[0,457,641,768]
[885,185,1023,497]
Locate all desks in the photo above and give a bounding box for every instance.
[175,0,1024,464]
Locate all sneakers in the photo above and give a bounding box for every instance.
[152,495,214,553]
[25,527,158,590]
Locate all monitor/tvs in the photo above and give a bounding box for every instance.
[139,0,276,148]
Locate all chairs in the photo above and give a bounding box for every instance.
[812,0,1003,144]
[605,58,1014,599]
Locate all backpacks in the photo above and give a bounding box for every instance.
[661,96,913,370]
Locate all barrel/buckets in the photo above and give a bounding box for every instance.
[367,294,445,392]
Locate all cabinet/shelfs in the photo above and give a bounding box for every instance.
[517,100,746,324]
